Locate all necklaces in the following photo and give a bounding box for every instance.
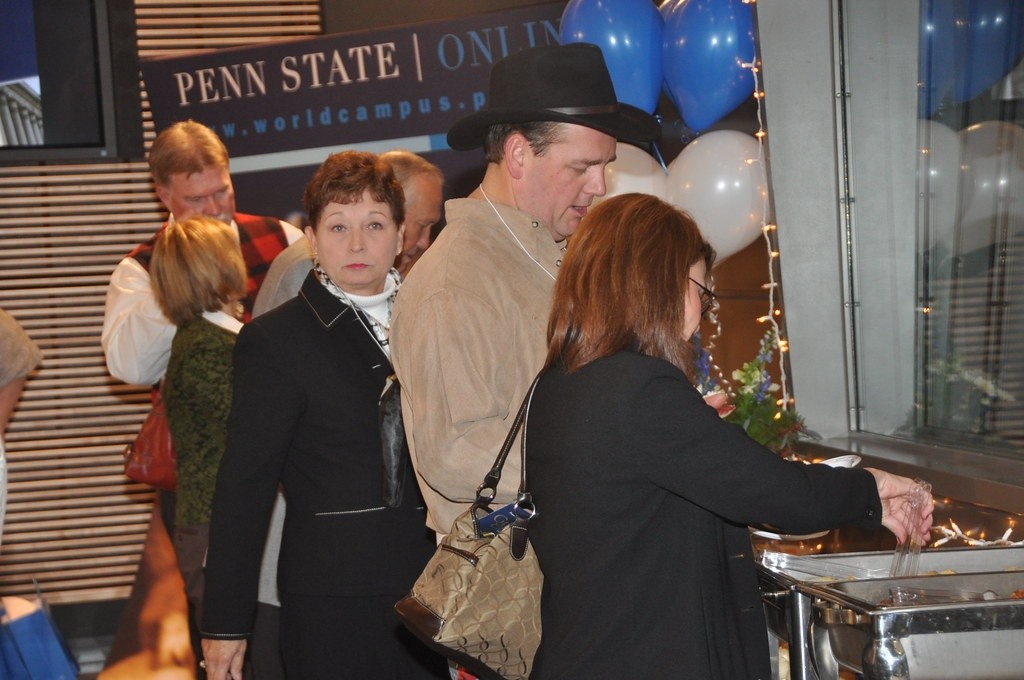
[334,282,392,367]
[479,184,558,283]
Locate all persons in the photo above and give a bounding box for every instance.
[199,151,453,680]
[520,194,936,680]
[102,121,309,546]
[149,217,244,679]
[0,309,44,537]
[390,37,728,680]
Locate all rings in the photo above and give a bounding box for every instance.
[912,502,916,508]
[199,660,206,669]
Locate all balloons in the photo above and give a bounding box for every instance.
[658,0,756,133]
[558,0,666,118]
[916,1,1024,264]
[580,139,668,223]
[668,129,768,270]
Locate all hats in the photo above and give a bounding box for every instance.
[446,43,660,150]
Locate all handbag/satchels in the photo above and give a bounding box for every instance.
[0,576,82,680]
[393,364,548,680]
[124,392,177,492]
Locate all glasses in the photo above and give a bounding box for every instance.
[689,278,717,315]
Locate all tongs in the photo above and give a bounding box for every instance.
[755,548,888,579]
[888,586,1000,607]
[889,478,931,577]
